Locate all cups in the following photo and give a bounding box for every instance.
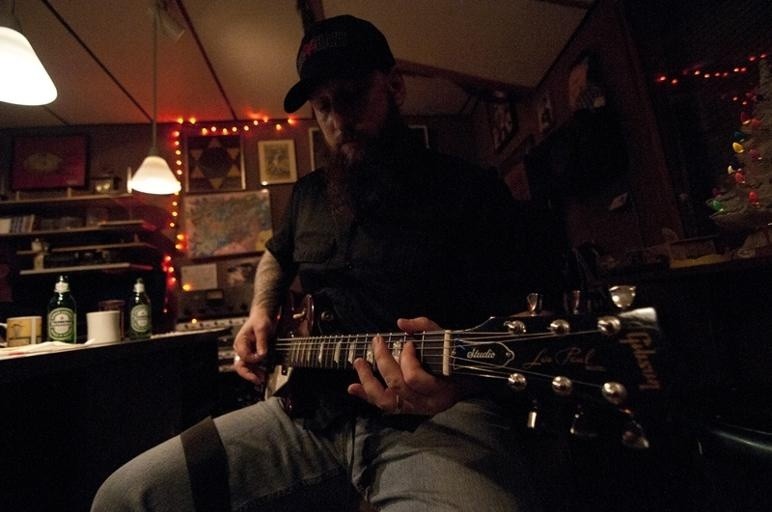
[85,310,121,344]
[0,316,42,348]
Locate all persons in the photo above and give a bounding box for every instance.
[0,241,31,341]
[84,16,553,512]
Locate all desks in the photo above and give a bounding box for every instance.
[3,328,232,508]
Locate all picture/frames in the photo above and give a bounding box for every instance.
[7,135,92,189]
[182,128,322,194]
[481,86,519,154]
[407,123,430,149]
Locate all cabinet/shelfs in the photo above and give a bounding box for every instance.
[1,192,161,276]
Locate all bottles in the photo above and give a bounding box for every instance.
[44,273,77,345]
[127,276,152,342]
[97,298,126,341]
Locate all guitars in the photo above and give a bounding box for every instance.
[264,286,674,462]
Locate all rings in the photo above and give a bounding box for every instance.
[392,395,403,416]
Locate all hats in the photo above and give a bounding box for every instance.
[284,15,396,114]
